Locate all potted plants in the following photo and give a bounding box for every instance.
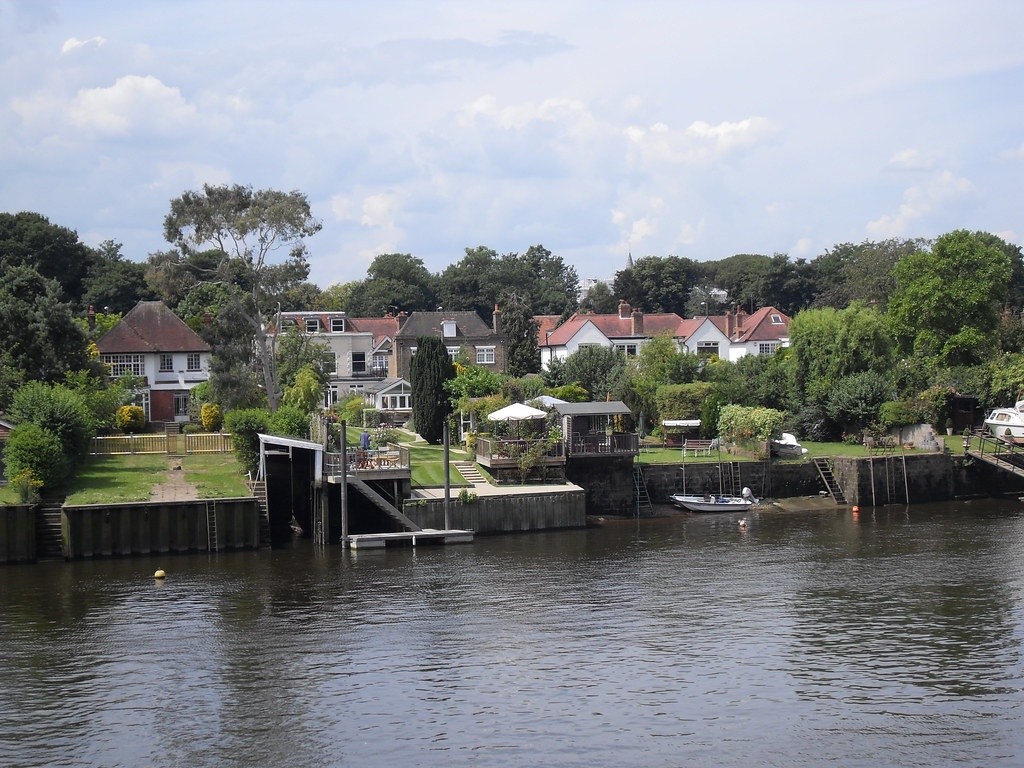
[1004,428,1013,446]
[945,417,953,436]
[962,428,972,447]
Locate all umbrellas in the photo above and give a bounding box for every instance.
[487,402,547,440]
[524,395,569,438]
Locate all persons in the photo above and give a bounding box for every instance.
[359,429,371,457]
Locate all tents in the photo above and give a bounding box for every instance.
[771,433,802,456]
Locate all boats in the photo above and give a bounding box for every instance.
[672,487,760,513]
[986,399,1024,444]
[771,432,802,458]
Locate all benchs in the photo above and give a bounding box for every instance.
[868,437,895,454]
[684,439,714,457]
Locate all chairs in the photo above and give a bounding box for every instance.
[496,436,536,459]
[349,445,400,470]
[573,432,608,452]
